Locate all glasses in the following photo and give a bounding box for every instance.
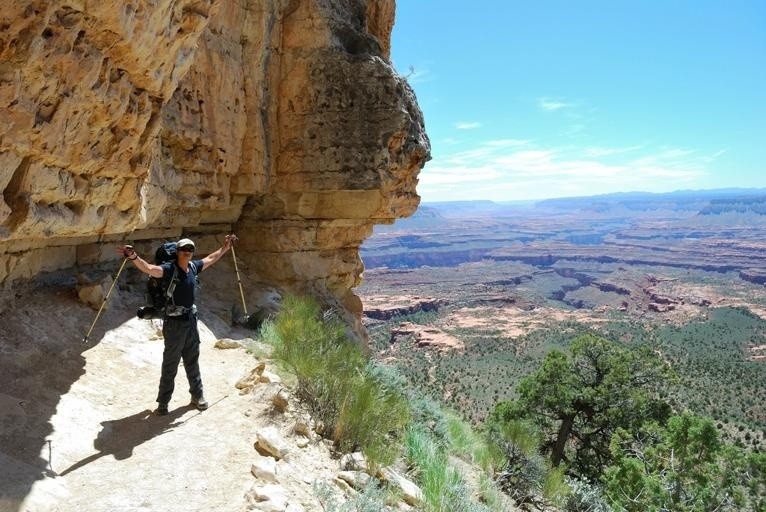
[178,246,195,252]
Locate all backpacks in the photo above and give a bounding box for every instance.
[147,241,197,311]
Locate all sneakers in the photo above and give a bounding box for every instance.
[190,395,208,410]
[157,402,168,415]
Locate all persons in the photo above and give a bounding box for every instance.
[115,231,240,416]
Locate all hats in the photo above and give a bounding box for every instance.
[176,238,196,249]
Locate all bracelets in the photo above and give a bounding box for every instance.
[128,253,138,262]
